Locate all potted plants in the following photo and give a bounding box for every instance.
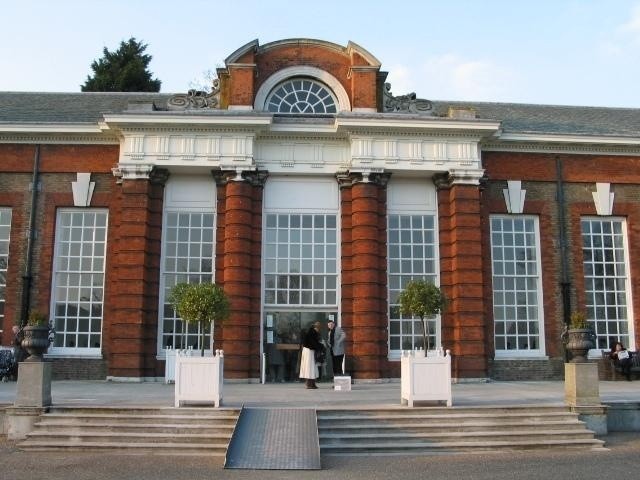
[395,280,452,406]
[19,310,57,362]
[165,283,198,384]
[561,311,597,363]
[175,282,230,407]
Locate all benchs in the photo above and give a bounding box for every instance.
[0,350,14,381]
[601,350,639,381]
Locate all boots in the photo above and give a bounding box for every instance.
[305,379,317,389]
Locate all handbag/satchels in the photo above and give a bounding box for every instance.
[315,350,325,363]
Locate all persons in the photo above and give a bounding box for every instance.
[608,342,632,381]
[299,321,325,389]
[326,320,346,375]
[12,325,26,382]
